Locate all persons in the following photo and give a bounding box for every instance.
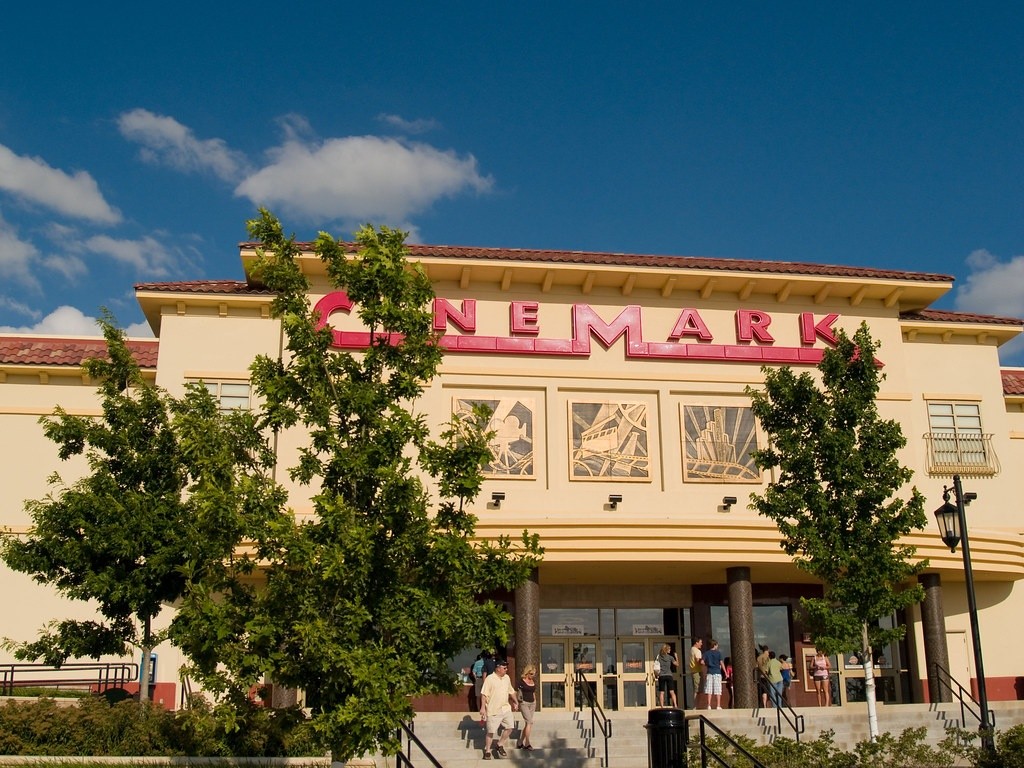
[756,648,792,709]
[655,644,680,710]
[700,640,733,710]
[480,658,520,760]
[689,635,705,710]
[809,643,831,707]
[512,664,538,753]
[472,650,496,712]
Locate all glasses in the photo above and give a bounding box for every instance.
[529,673,535,675]
[499,667,508,670]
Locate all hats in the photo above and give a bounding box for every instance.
[495,659,509,668]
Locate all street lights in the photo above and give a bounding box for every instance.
[932,471,998,767]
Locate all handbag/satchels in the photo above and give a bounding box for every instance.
[517,687,523,702]
[780,667,791,686]
[653,654,660,676]
[809,657,815,676]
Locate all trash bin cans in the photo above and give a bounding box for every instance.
[645,709,688,768]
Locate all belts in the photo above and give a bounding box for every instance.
[477,676,482,678]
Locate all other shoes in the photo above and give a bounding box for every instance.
[717,707,723,710]
[708,705,711,709]
[495,743,507,756]
[484,749,491,759]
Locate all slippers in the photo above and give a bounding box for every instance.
[523,745,534,751]
[517,741,524,749]
